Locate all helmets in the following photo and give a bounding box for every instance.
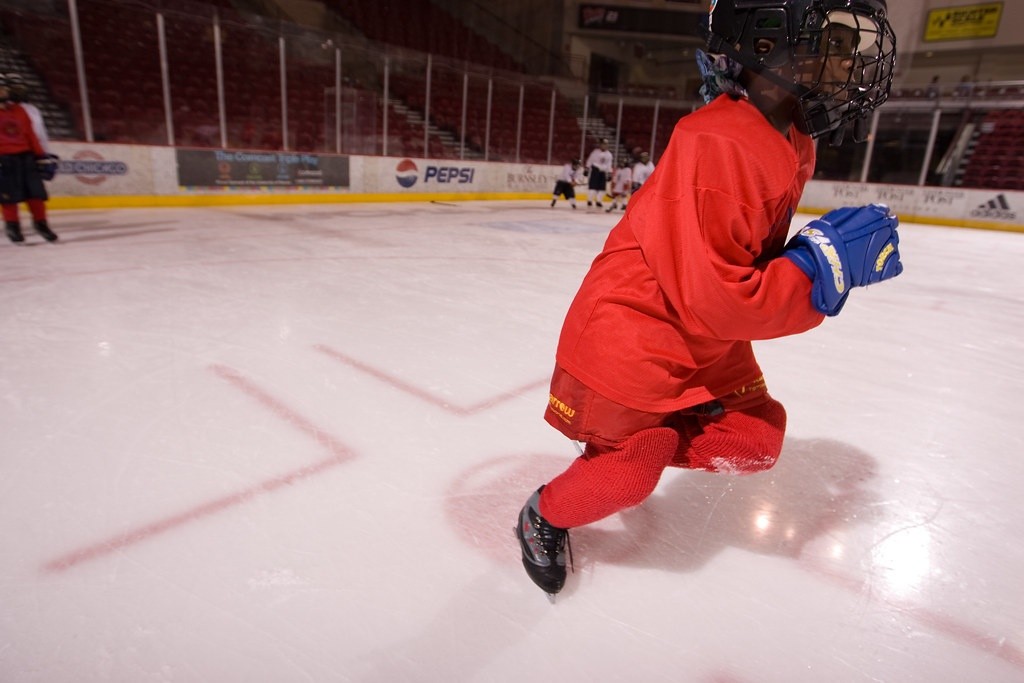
[639,151,649,157]
[705,1,888,65]
[571,157,582,164]
[0,74,12,87]
[618,157,627,162]
[600,138,608,145]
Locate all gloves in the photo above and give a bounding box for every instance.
[37,158,55,180]
[783,203,903,315]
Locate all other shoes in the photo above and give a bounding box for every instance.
[588,201,592,206]
[34,220,57,242]
[622,204,626,209]
[613,202,616,207]
[573,204,576,209]
[5,222,23,244]
[596,201,601,207]
[551,202,555,207]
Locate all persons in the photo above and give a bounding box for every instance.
[0,71,60,247]
[926,74,941,100]
[953,74,970,98]
[518,0,905,595]
[583,137,613,207]
[551,156,583,209]
[631,151,656,194]
[606,158,632,212]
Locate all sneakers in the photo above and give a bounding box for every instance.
[513,485,574,604]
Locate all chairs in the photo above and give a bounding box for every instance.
[890,85,1023,191]
[0,0,695,168]
